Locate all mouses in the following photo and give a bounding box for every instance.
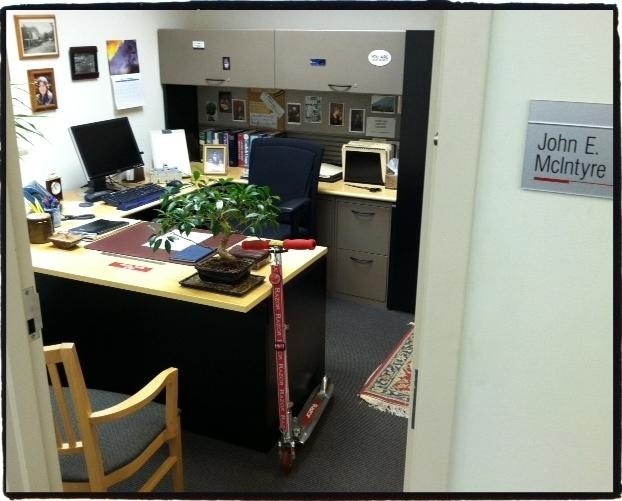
[167,180,183,188]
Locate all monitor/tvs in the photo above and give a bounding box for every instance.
[68,116,145,194]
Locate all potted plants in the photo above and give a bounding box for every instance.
[145,169,284,284]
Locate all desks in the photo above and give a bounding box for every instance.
[25,160,398,453]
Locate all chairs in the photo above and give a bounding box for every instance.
[242,139,324,239]
[42,341,185,492]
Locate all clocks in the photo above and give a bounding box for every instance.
[44,175,63,201]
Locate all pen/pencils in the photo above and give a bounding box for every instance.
[42,196,57,208]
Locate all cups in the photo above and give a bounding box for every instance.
[26,213,53,244]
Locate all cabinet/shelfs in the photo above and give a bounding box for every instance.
[275,29,406,98]
[152,27,275,91]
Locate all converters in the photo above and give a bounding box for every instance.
[85,189,111,202]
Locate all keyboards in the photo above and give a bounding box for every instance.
[102,181,170,211]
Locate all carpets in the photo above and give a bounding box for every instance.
[358,322,415,420]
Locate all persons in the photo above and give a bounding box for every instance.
[207,148,224,173]
[36,75,55,105]
[330,106,343,125]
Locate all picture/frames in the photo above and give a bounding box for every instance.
[24,66,59,116]
[205,141,228,174]
[69,44,100,82]
[12,13,64,61]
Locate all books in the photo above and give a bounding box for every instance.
[68,219,129,235]
[170,243,218,262]
[199,126,287,168]
[70,217,143,242]
